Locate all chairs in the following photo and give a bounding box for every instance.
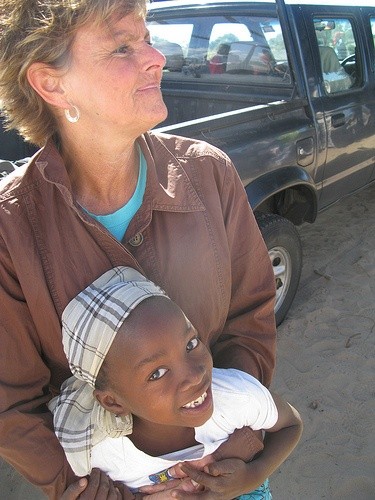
[318,45,345,74]
[152,42,185,72]
[226,41,275,75]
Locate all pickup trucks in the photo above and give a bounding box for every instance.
[0,0,375,329]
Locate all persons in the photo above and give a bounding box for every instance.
[0,0,279,499]
[331,31,349,61]
[44,265,303,500]
